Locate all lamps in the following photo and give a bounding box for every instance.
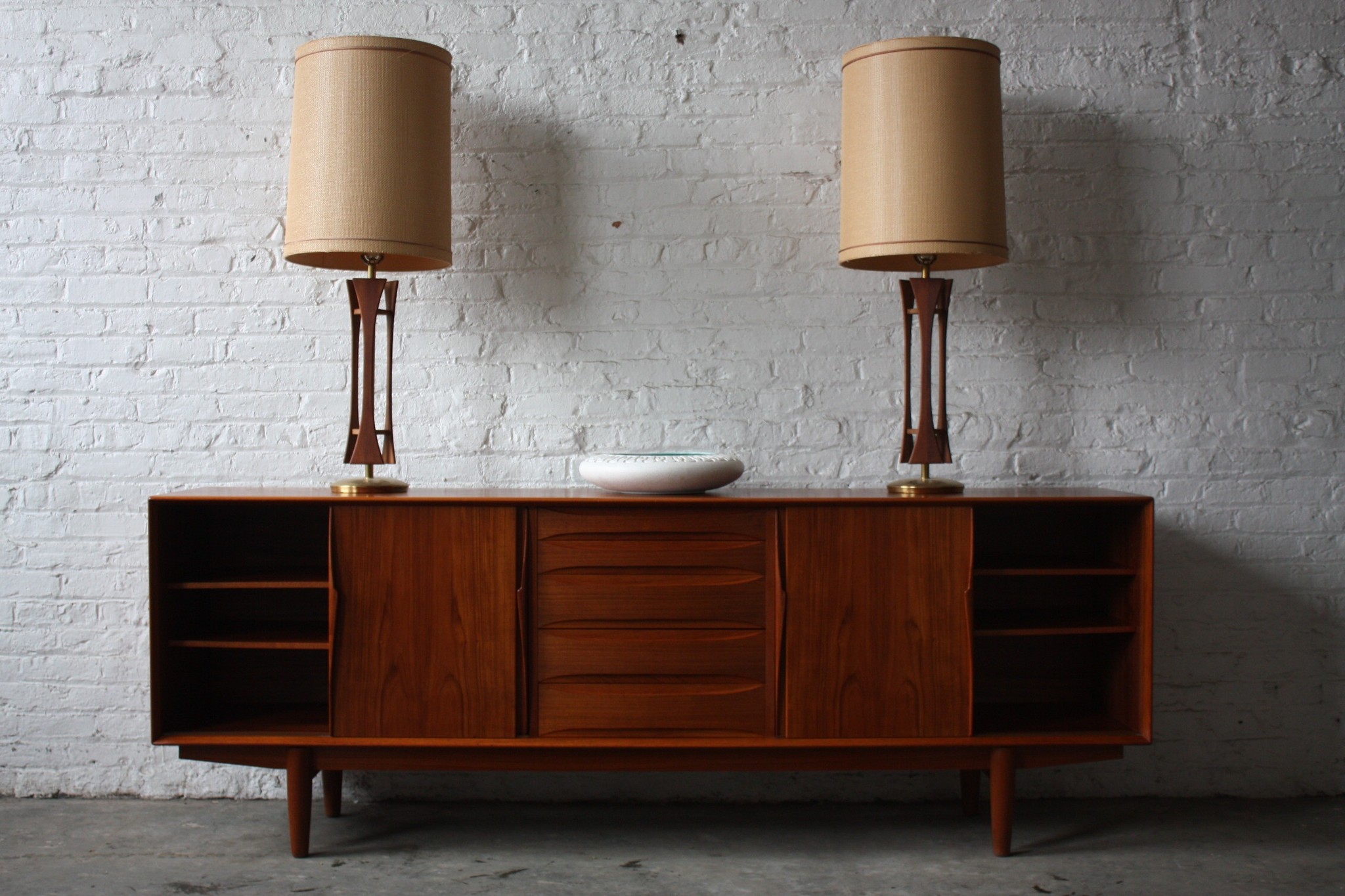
[284,36,452,493]
[838,36,1008,495]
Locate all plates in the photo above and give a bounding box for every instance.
[580,451,745,495]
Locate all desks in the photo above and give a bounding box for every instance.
[148,491,1155,858]
